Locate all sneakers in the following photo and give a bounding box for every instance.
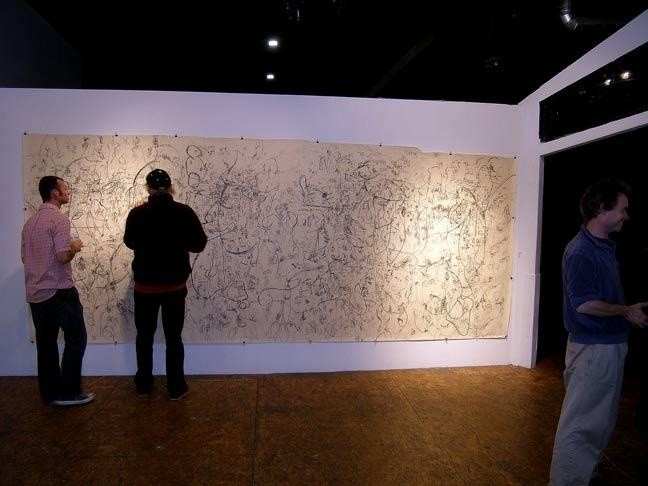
[47,392,97,405]
[170,384,189,400]
[135,383,154,396]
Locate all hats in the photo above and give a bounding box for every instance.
[146,169,170,190]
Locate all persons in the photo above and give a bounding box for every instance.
[20,176,96,405]
[547,181,648,485]
[123,169,208,401]
